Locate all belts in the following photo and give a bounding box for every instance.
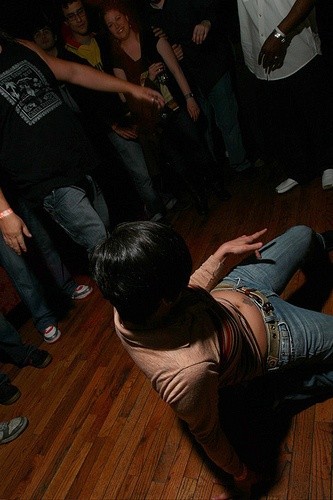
[210,283,279,371]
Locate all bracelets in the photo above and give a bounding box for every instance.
[234,464,248,481]
[273,27,289,44]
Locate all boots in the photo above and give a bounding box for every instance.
[182,162,230,216]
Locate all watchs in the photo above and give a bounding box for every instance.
[185,93,194,100]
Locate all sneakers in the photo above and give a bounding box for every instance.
[0,415,27,443]
[27,348,51,368]
[161,192,177,210]
[276,178,298,193]
[72,285,93,299]
[0,382,21,404]
[41,325,61,343]
[322,169,333,189]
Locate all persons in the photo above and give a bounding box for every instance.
[144,0,255,184]
[101,6,231,215]
[0,312,53,404]
[61,0,179,221]
[1,415,29,445]
[31,20,69,61]
[0,187,93,343]
[91,221,333,499]
[238,0,333,195]
[0,32,164,246]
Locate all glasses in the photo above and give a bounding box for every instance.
[64,8,84,19]
